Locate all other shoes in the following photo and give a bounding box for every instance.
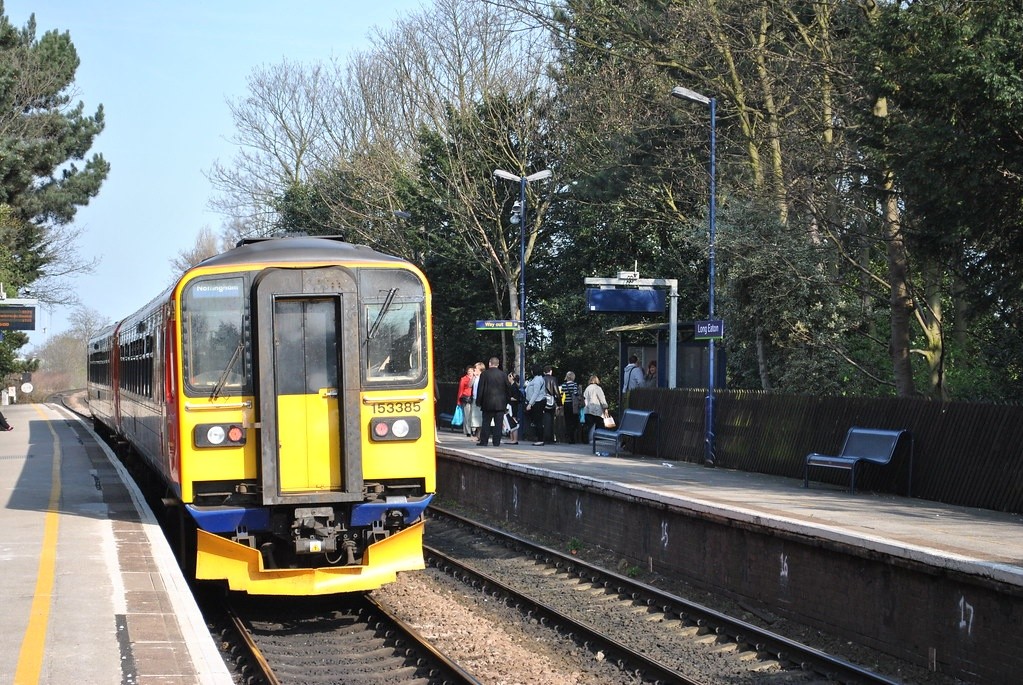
[504,442,518,445]
[476,443,487,446]
[532,443,544,446]
[435,440,441,443]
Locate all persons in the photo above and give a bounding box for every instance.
[645,360,657,387]
[583,375,609,444]
[434,379,442,444]
[526,365,562,445]
[457,357,521,446]
[0,412,13,430]
[622,356,645,394]
[561,370,581,443]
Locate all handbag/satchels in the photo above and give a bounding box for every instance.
[603,415,616,429]
[451,405,464,426]
[579,407,585,424]
[572,395,585,414]
[460,394,472,404]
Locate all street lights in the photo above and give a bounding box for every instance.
[493,169,553,441]
[671,84,718,468]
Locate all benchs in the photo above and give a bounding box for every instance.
[439,413,463,432]
[593,408,660,458]
[804,425,915,496]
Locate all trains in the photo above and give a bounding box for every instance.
[87,234,438,598]
